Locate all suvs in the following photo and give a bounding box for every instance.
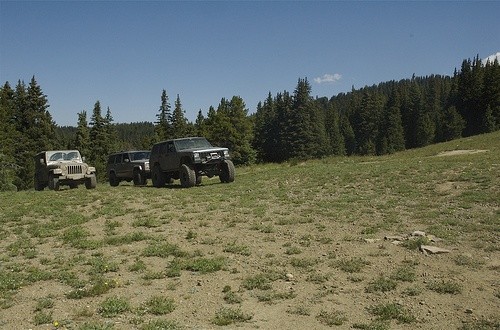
[148,137,234,188]
[34,149,96,190]
[107,150,155,186]
